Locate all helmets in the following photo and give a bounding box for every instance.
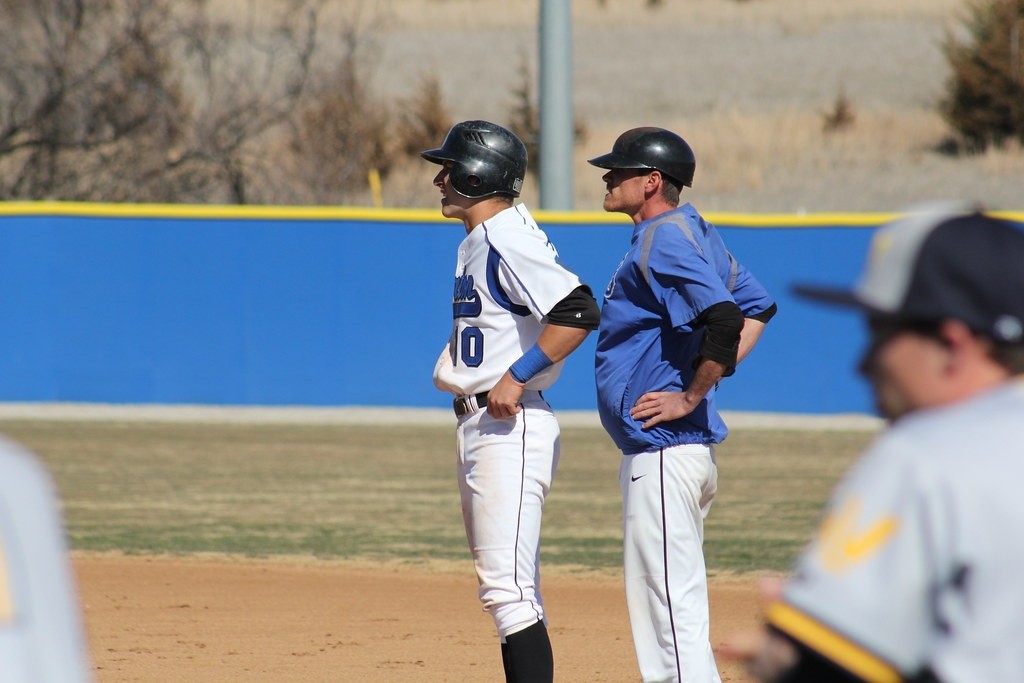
[587,126,696,188]
[421,120,528,198]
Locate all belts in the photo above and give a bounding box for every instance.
[453,388,544,417]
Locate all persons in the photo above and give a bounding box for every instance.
[716,209,1024,683]
[419,120,601,683]
[586,127,777,683]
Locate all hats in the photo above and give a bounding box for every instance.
[796,203,1023,353]
